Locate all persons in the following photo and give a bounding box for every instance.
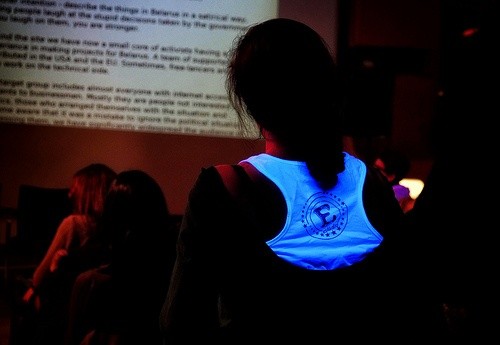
[25,163,122,304]
[367,138,415,213]
[157,18,408,344]
[50,170,180,345]
